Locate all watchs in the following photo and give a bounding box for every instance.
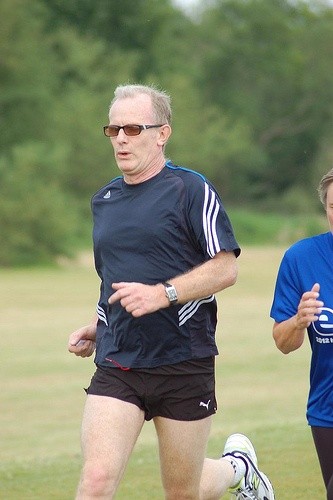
[162,280,178,308]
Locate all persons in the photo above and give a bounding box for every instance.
[271,166,332,500]
[68,79,276,500]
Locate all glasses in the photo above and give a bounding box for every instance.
[103,123,164,137]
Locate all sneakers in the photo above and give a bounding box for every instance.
[221,433,275,500]
